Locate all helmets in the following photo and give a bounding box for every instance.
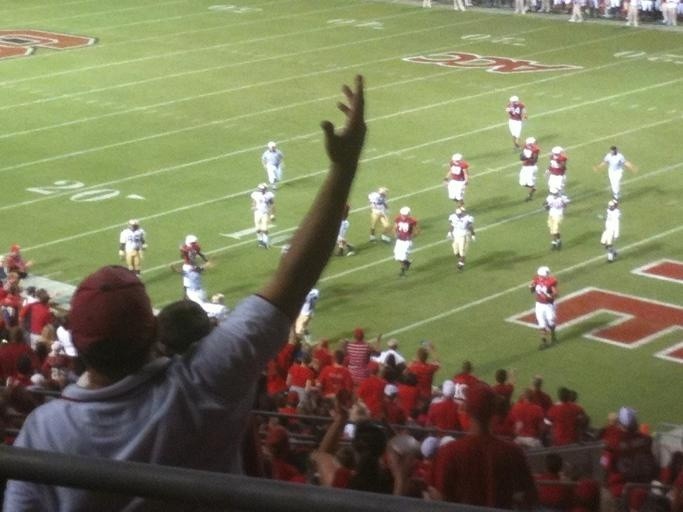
[185,235,198,248]
[257,183,267,192]
[129,219,139,231]
[268,142,276,152]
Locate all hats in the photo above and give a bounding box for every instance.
[153,300,216,356]
[71,267,155,363]
[12,245,21,251]
[618,407,637,430]
[267,328,458,457]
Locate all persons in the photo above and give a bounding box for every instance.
[392,206,419,276]
[2,73,366,510]
[367,186,392,244]
[335,206,357,257]
[262,142,284,191]
[0,245,682,510]
[179,234,208,269]
[422,0,683,27]
[546,145,567,194]
[505,96,528,146]
[517,136,541,203]
[592,145,636,203]
[545,187,570,247]
[600,199,621,263]
[118,218,145,276]
[443,204,475,270]
[444,153,471,211]
[250,181,276,249]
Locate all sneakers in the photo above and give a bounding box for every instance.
[421,0,679,27]
